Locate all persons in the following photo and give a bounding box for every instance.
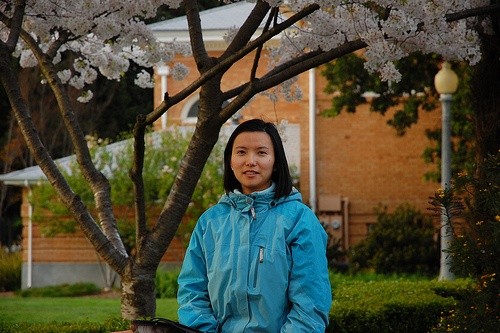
[176,119,332,333]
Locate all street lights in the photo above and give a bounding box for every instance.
[434,61,459,281]
